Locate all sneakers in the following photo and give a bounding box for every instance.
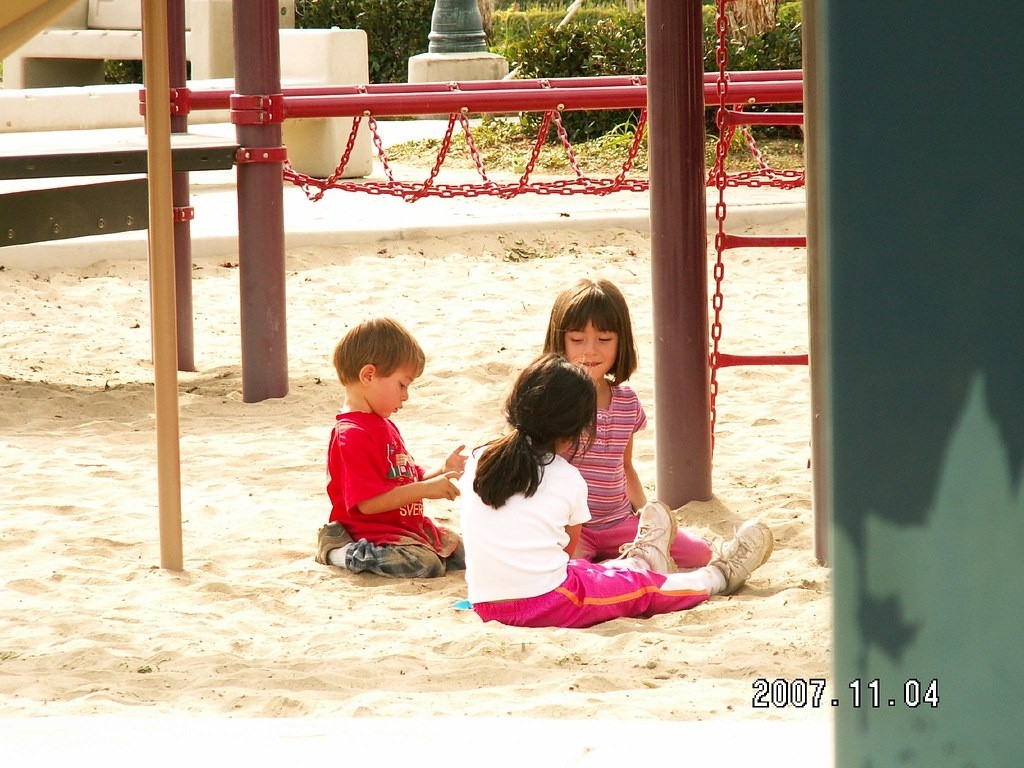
[616,499,679,574]
[315,521,354,565]
[707,517,773,596]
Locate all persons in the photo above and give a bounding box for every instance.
[459,352,774,628]
[542,277,713,568]
[314,316,469,579]
